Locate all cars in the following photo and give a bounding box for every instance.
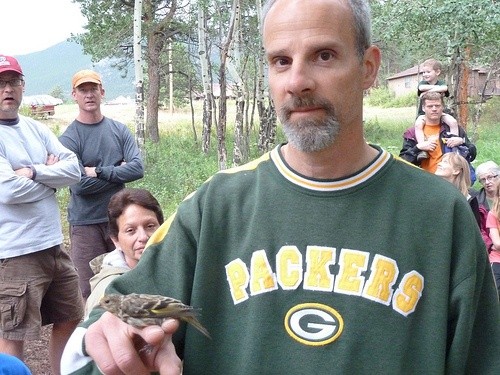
[23,95,64,120]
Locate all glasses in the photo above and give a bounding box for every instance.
[0,79,22,88]
[479,175,497,184]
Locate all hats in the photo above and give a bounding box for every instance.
[0,55,23,77]
[72,70,101,88]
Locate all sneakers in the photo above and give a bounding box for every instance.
[416,150,430,161]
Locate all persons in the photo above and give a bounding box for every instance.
[82,186,166,323]
[0,48,85,375]
[58,1,500,375]
[397,57,499,291]
[57,69,147,317]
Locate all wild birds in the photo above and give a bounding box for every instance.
[92,293,212,355]
